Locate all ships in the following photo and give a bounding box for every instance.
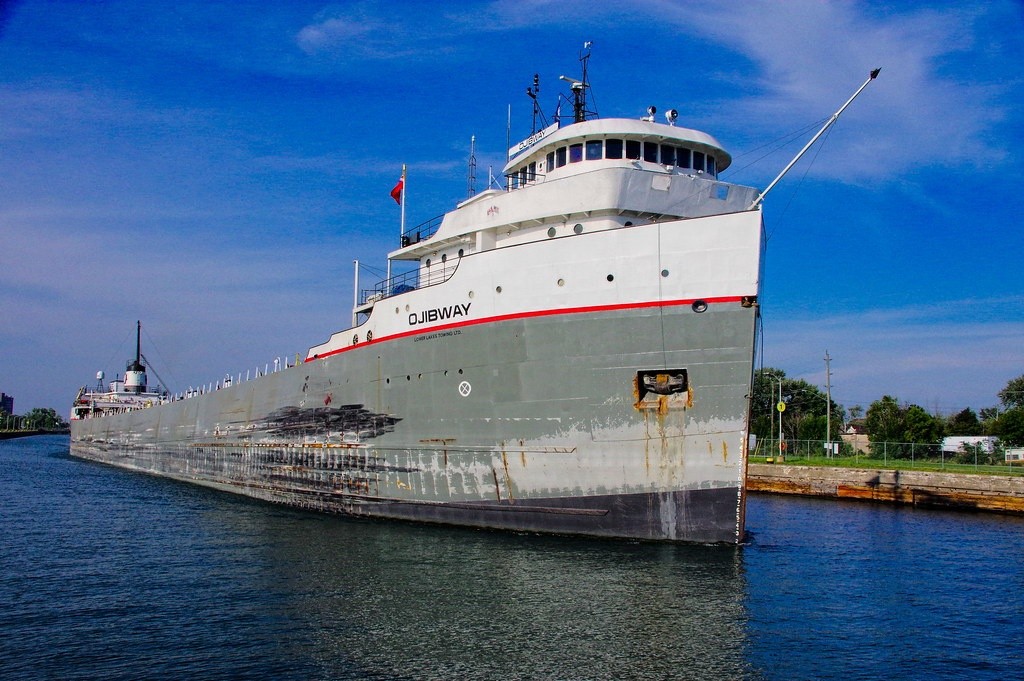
[69,34,880,548]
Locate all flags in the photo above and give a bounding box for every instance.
[390,175,404,205]
